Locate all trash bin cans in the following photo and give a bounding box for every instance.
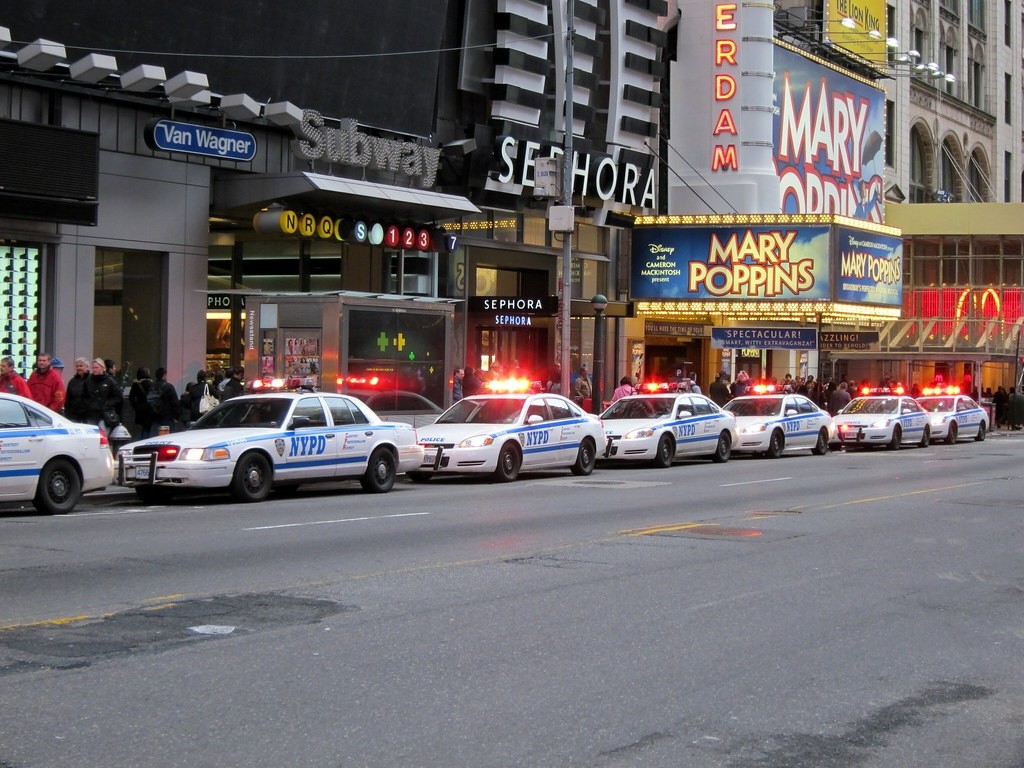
[981,402,996,431]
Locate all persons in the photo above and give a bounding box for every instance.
[0,356,34,401]
[263,338,319,373]
[859,379,1024,429]
[27,352,67,412]
[547,367,592,399]
[49,357,182,460]
[688,371,701,393]
[771,373,858,415]
[453,358,535,404]
[708,369,750,407]
[610,376,638,406]
[179,366,245,429]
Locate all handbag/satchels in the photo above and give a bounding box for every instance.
[199,384,219,413]
[104,410,119,426]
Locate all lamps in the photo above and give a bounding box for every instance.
[774,11,955,81]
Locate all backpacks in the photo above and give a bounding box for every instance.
[146,382,168,414]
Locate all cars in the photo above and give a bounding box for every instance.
[114,392,426,502]
[0,390,114,516]
[597,392,737,469]
[916,395,990,444]
[720,393,833,458]
[340,390,444,427]
[404,392,608,484]
[833,395,932,451]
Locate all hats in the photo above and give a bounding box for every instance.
[51,358,65,368]
